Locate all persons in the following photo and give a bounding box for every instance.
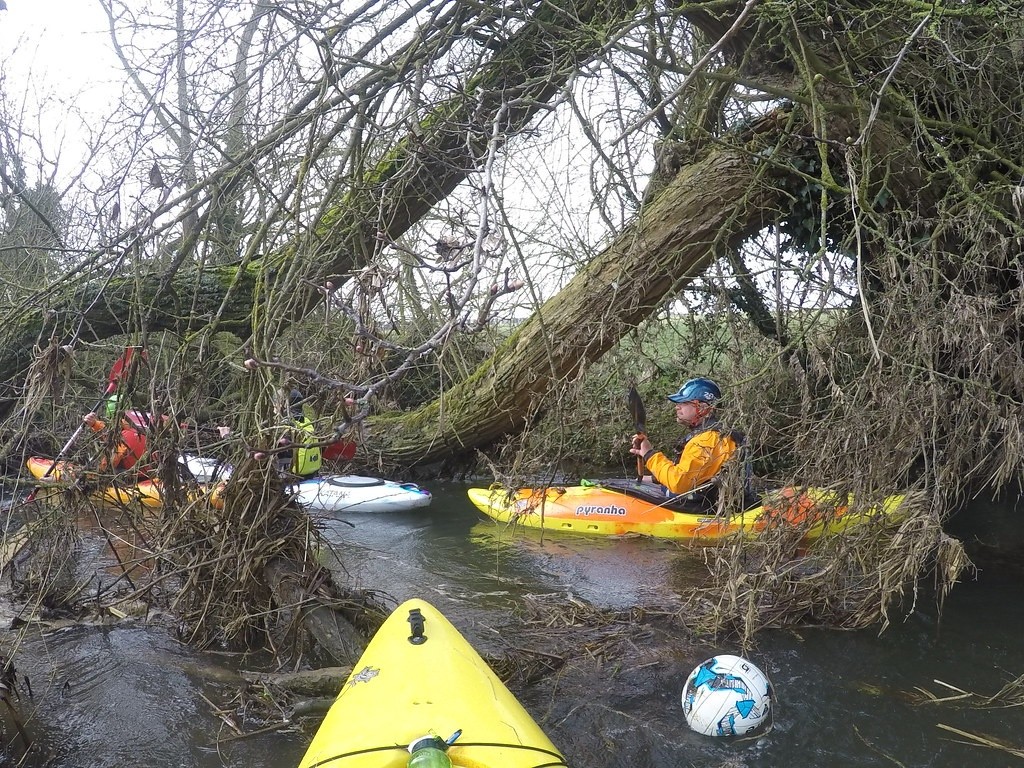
[85,395,150,486]
[320,398,359,464]
[258,389,322,482]
[629,378,737,515]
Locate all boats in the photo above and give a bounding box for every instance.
[178,455,431,511]
[468,477,914,542]
[27,454,227,510]
[121,409,357,461]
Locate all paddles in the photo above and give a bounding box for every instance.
[25,344,149,504]
[629,386,647,484]
[126,408,357,462]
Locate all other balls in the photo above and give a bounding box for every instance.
[679,654,772,738]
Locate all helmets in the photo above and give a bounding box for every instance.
[273,387,304,407]
[667,376,722,407]
[106,394,132,419]
